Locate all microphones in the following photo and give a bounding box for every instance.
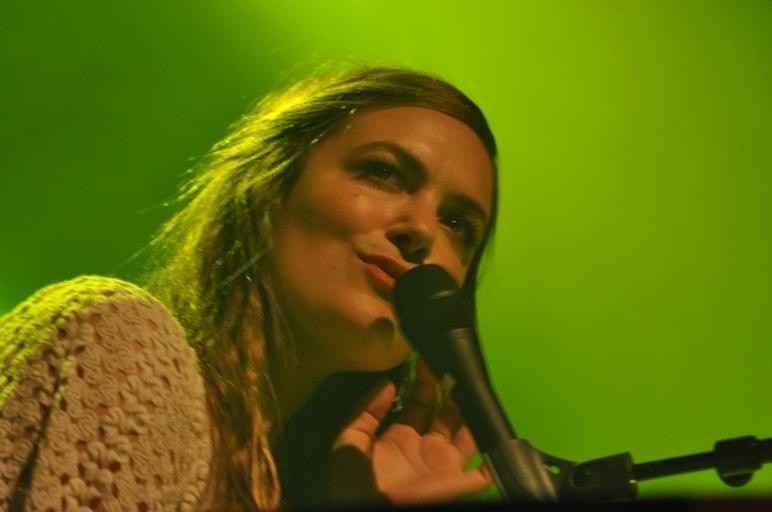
[390,263,576,507]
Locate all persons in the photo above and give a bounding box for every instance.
[0,64,501,512]
